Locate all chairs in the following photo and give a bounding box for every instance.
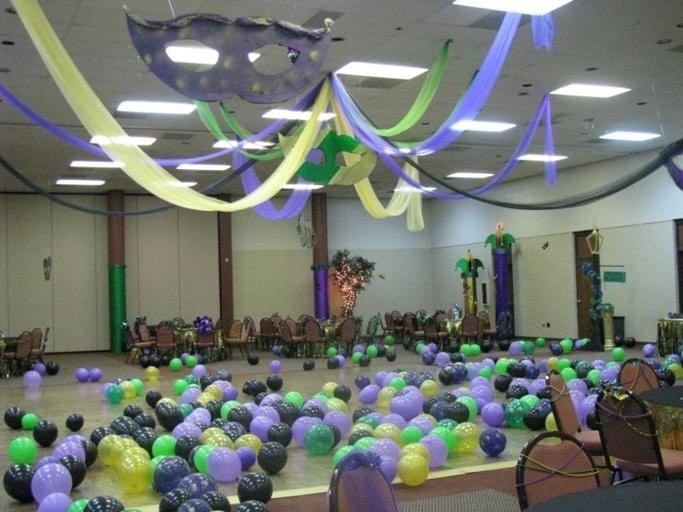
[0,327,50,380]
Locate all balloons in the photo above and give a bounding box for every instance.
[403,337,594,368]
[262,365,479,487]
[2,352,273,512]
[466,337,683,455]
[268,336,396,373]
